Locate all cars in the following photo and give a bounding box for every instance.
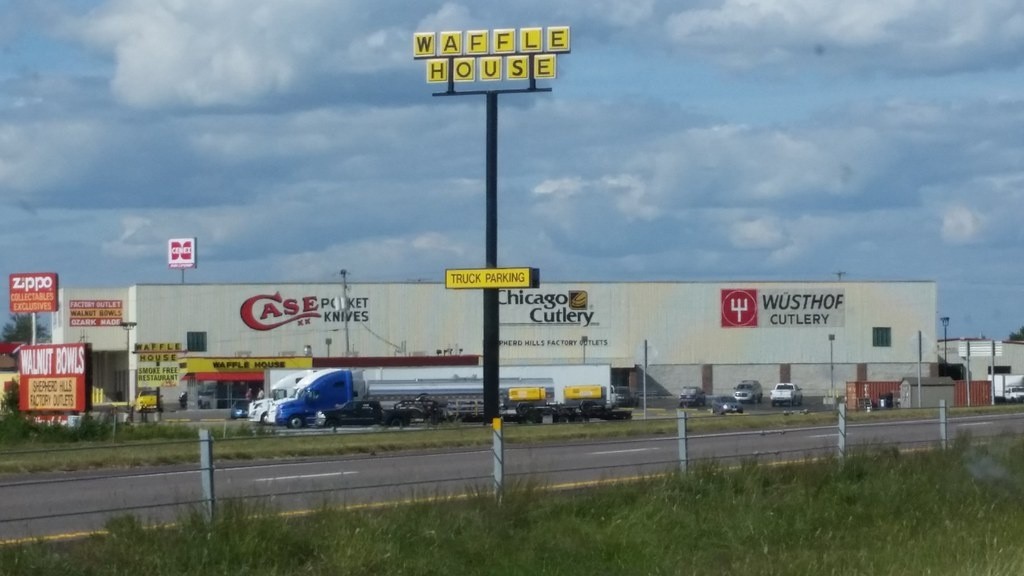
[230,398,251,420]
[179,382,245,409]
[136,390,165,413]
[679,386,706,406]
[712,396,744,415]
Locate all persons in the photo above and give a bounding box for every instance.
[246,389,253,400]
[257,387,264,400]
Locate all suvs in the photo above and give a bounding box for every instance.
[1004,385,1024,404]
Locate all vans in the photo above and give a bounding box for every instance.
[733,380,763,405]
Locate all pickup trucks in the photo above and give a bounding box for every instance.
[770,382,803,407]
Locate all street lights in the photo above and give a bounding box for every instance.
[828,334,835,409]
[941,317,949,376]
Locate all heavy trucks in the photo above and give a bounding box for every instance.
[246,362,616,429]
